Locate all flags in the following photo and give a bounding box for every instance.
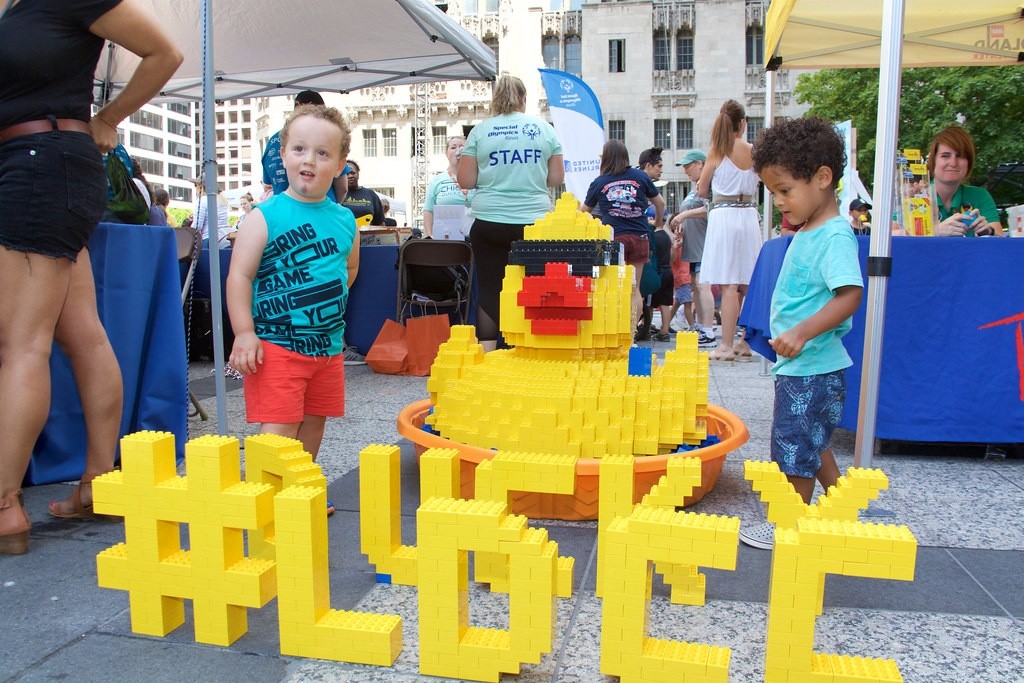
[539,67,606,207]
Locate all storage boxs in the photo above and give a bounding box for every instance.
[359,225,414,246]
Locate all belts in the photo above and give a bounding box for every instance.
[1,119,92,139]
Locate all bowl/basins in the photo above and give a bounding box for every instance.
[397,398,751,521]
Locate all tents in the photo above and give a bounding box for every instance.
[220,183,405,227]
[98,0,502,431]
[760,1,1024,471]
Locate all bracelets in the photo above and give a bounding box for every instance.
[94,114,118,133]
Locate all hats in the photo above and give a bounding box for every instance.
[850,198,872,210]
[635,149,662,167]
[675,149,707,167]
[188,175,205,184]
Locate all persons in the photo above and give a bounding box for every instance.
[225,103,362,516]
[260,90,397,366]
[101,143,273,248]
[0,1,183,557]
[579,98,1006,362]
[737,106,887,553]
[427,76,565,355]
[412,228,422,239]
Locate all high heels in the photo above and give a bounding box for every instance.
[48,467,124,523]
[0,490,32,555]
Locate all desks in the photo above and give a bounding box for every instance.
[30,218,189,490]
[200,242,480,358]
[735,234,1024,456]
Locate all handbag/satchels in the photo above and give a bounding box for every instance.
[364,300,451,377]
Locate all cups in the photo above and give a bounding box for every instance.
[1005,204,1024,238]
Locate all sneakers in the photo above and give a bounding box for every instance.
[698,328,717,347]
[343,346,367,365]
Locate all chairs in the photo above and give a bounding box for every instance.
[396,237,474,325]
[174,226,209,422]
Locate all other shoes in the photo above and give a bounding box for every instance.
[636,332,651,341]
[740,521,775,550]
[707,342,753,366]
[653,332,671,342]
